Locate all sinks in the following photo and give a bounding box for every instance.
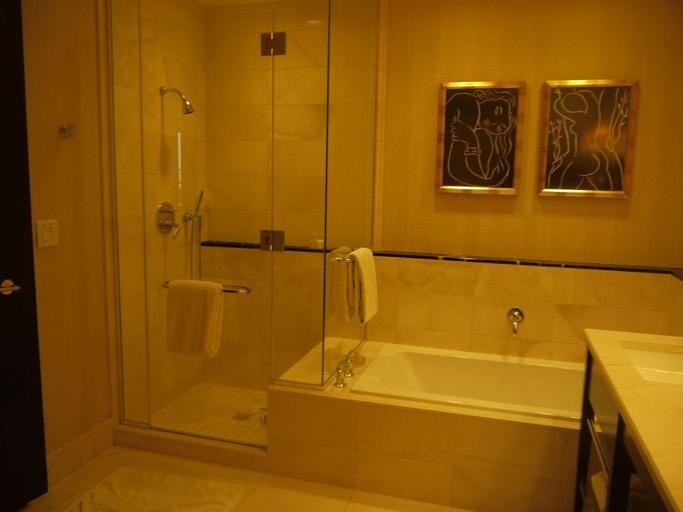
[623,339,683,383]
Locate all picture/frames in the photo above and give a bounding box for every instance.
[535,78,640,198]
[435,80,527,196]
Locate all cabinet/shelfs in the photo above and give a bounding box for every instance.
[572,350,666,511]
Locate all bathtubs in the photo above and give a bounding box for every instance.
[350,346,589,422]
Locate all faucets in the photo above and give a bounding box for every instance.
[507,308,525,336]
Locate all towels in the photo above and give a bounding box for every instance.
[327,245,350,324]
[344,247,379,325]
[165,279,224,359]
